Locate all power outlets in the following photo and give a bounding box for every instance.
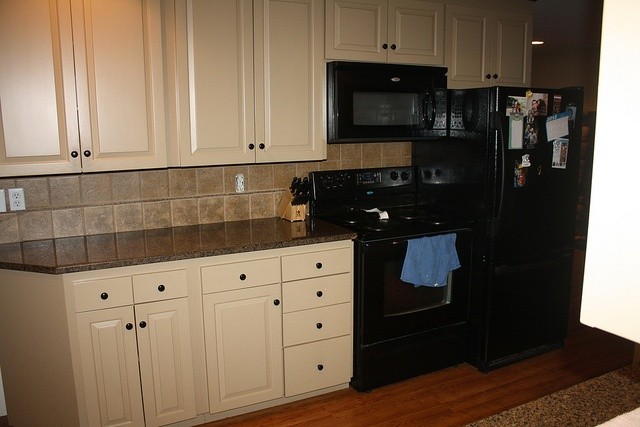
[8,187,26,211]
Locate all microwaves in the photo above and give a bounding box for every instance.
[327,61,449,145]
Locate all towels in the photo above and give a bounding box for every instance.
[400,232,462,289]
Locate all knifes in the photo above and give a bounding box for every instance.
[289,176,312,205]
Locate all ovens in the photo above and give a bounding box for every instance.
[350,228,473,392]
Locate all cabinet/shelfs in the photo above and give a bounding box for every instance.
[1,0,162,179]
[199,242,357,424]
[446,2,534,90]
[324,0,445,67]
[0,259,203,427]
[162,0,327,168]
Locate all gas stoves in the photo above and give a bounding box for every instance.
[320,204,474,244]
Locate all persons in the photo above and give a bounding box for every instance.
[525,124,537,144]
[568,109,574,128]
[531,98,546,115]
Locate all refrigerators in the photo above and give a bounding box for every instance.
[413,85,577,374]
